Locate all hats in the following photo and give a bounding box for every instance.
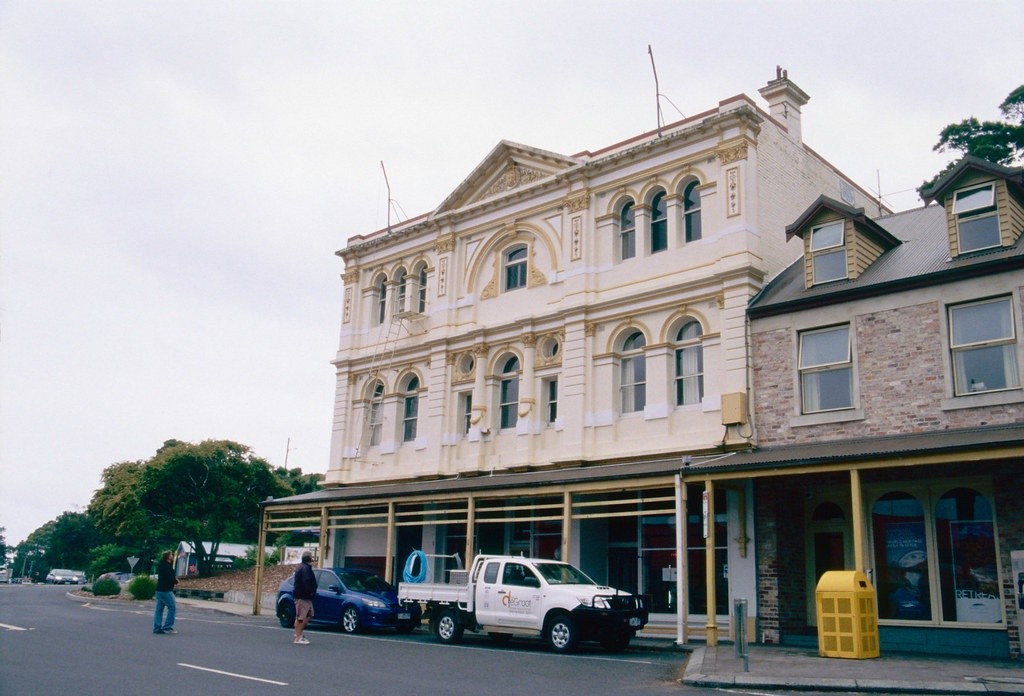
[302,551,312,558]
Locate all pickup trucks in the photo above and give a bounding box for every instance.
[400,553,650,654]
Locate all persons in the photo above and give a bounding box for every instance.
[292,551,318,645]
[153,550,179,634]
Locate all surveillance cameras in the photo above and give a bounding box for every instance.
[683,456,692,466]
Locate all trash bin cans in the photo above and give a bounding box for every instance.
[815,571,879,659]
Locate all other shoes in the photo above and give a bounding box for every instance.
[294,639,310,644]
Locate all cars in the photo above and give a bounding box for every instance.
[72,573,87,585]
[275,567,422,636]
[46,568,80,586]
[97,571,136,582]
[0,566,9,583]
